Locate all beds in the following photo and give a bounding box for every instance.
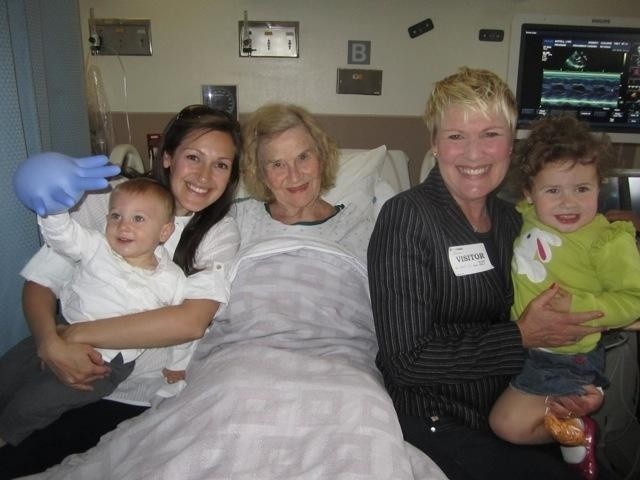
[12,144,449,480]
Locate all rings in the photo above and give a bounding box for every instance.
[568,411,575,418]
[568,335,577,341]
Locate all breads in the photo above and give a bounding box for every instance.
[545,413,584,446]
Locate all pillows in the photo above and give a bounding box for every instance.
[232,145,387,220]
[374,154,401,220]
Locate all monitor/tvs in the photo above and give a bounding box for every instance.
[506,13,640,146]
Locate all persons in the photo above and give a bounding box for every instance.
[487,108,639,480]
[366,68,639,480]
[223,102,374,273]
[0,106,247,480]
[0,176,198,451]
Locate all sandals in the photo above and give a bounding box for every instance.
[561,416,598,480]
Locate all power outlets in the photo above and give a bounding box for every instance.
[88,19,153,56]
